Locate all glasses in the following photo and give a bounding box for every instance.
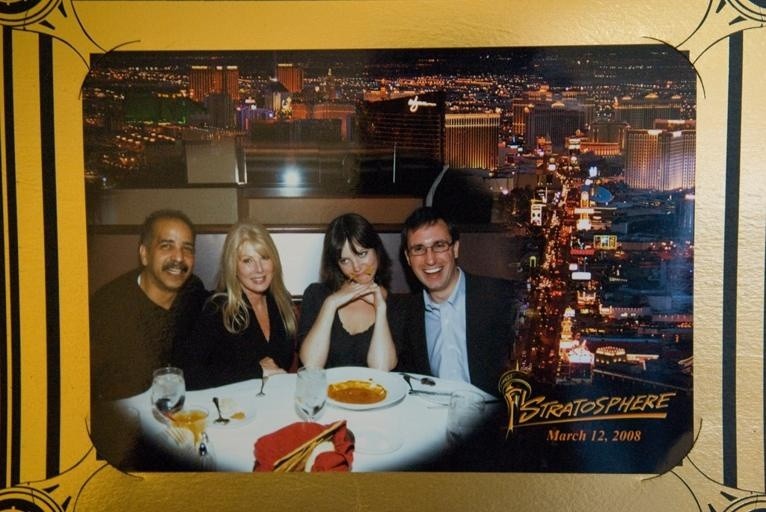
[409,241,454,256]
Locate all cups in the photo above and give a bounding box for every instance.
[448,390,485,442]
[151,367,209,467]
[296,365,327,422]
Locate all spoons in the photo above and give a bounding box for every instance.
[212,396,230,425]
[400,372,435,386]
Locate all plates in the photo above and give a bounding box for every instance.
[323,366,406,410]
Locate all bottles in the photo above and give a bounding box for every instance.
[195,432,217,472]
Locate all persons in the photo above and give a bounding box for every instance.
[394,203,529,472]
[87,207,205,473]
[165,218,300,472]
[300,213,418,370]
